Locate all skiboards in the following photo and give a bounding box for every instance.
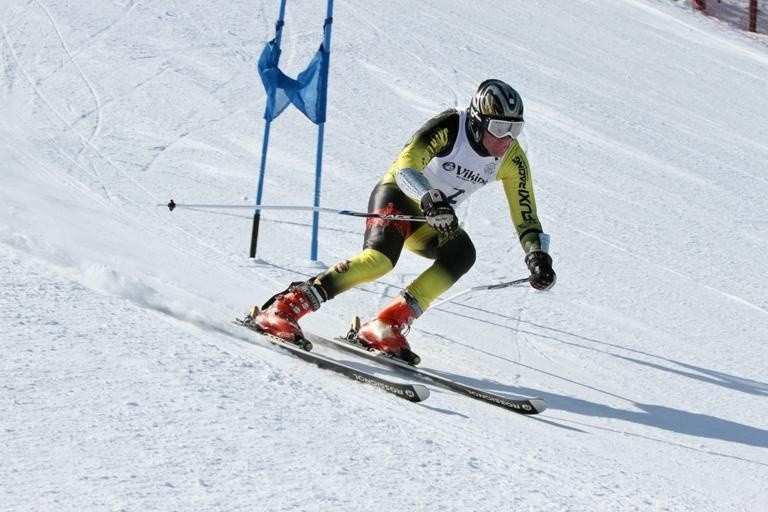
[109,266,548,414]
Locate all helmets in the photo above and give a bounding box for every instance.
[469,78,524,125]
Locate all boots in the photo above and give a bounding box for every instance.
[355,288,424,365]
[250,276,330,352]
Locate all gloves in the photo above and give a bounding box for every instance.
[524,250,556,292]
[419,187,458,235]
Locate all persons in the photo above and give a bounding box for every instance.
[248,78,557,364]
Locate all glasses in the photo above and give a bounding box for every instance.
[470,104,525,140]
